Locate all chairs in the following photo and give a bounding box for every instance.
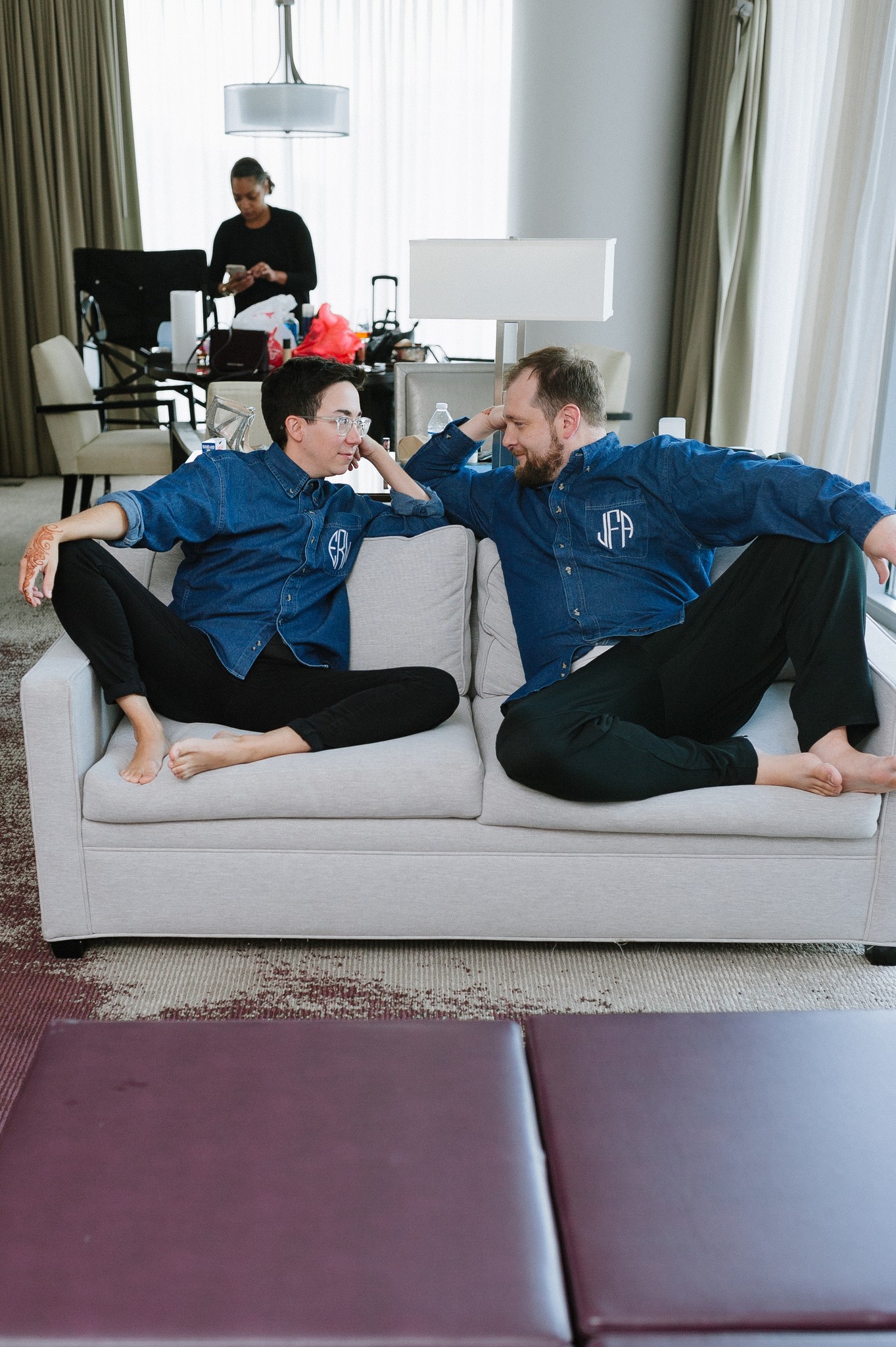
[31,334,181,529]
[69,243,211,431]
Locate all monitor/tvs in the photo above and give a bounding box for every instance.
[209,329,269,373]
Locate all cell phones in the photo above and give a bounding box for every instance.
[223,263,247,284]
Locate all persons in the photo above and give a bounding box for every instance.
[18,355,463,788]
[402,342,896,804]
[200,154,319,336]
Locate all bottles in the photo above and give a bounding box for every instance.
[303,303,314,340]
[284,312,299,344]
[428,403,453,442]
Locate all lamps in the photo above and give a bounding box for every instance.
[219,2,346,136]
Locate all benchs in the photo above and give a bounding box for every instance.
[1,1016,896,1344]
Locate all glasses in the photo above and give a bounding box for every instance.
[280,415,372,438]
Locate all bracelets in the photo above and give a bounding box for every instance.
[220,283,230,297]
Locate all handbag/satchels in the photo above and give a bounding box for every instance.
[364,320,419,366]
[208,327,270,374]
[231,293,298,372]
[291,302,361,366]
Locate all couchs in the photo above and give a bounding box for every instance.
[21,497,896,958]
